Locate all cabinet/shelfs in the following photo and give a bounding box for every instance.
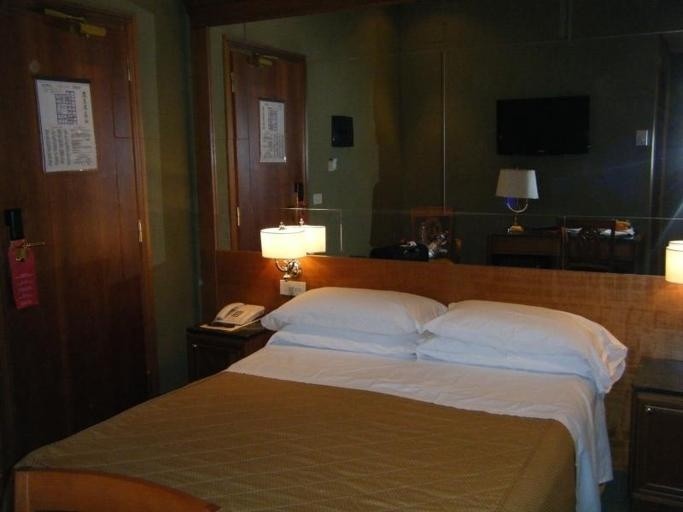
[482,225,646,274]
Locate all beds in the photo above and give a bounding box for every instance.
[12,346,602,512]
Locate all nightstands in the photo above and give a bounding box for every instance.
[624,356,682,512]
[186,325,274,382]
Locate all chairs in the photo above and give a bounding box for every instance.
[407,206,455,256]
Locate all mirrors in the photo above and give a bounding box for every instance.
[207,0,683,276]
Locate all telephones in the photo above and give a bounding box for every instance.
[213,303,265,325]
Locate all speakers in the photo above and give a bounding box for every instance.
[331,115,353,149]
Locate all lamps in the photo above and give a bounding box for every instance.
[302,225,326,254]
[665,247,683,289]
[260,224,306,281]
[494,168,539,232]
[669,240,683,247]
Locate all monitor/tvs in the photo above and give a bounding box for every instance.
[495,95,590,157]
